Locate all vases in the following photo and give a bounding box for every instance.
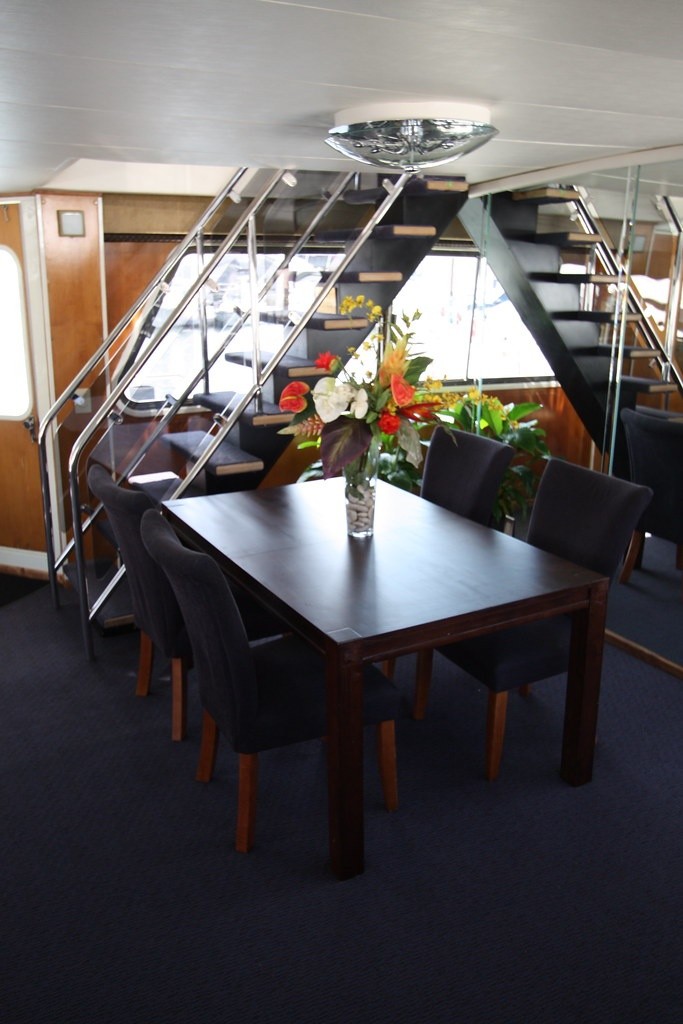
[276,292,448,545]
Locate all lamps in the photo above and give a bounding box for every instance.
[326,102,501,174]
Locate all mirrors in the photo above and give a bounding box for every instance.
[342,155,683,679]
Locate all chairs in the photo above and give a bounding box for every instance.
[83,462,281,742]
[137,508,414,859]
[413,456,653,783]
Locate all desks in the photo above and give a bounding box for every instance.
[161,470,614,883]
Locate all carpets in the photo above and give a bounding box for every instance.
[2,587,683,1023]
